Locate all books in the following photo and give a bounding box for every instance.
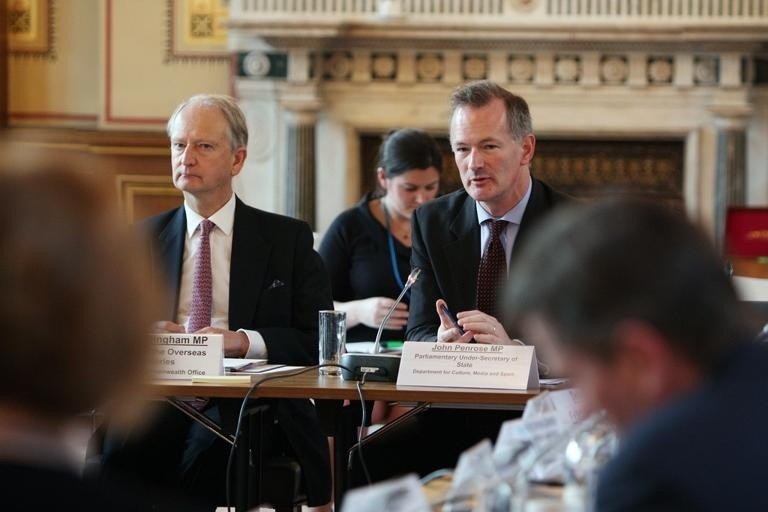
[224,356,270,373]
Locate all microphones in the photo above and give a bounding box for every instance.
[342,267,422,383]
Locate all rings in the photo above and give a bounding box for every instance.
[492,324,498,334]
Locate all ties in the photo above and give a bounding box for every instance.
[477,218,506,325]
[186,220,215,410]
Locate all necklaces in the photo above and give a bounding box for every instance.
[389,215,413,241]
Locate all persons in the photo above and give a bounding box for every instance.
[314,129,447,440]
[0,135,165,512]
[84,93,339,511]
[343,77,578,495]
[494,187,767,512]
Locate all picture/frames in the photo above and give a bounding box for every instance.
[163,1,233,64]
[2,1,58,62]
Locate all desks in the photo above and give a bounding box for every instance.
[133,359,576,512]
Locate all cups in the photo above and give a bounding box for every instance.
[319,310,347,376]
